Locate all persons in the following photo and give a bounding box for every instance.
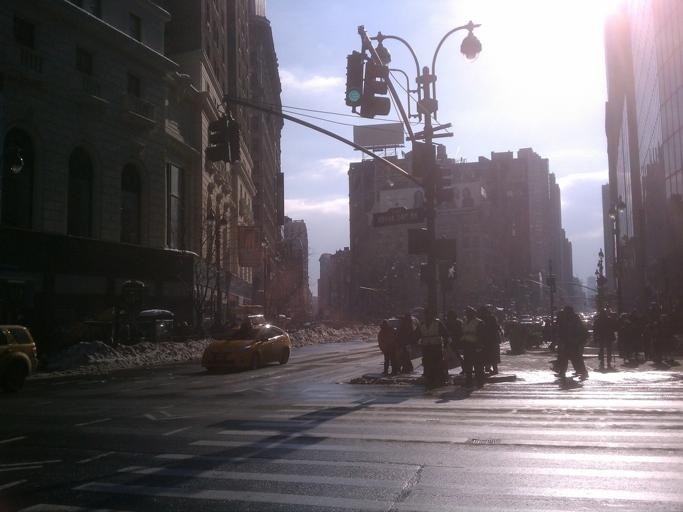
[376,300,503,387]
[548,305,589,383]
[597,308,618,371]
[411,185,475,210]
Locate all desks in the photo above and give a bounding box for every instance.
[607,193,631,319]
[592,248,606,311]
[204,182,234,319]
[257,237,275,319]
[369,21,490,388]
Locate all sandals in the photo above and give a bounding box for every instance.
[247,310,287,328]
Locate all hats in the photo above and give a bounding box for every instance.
[0,323,39,382]
[201,320,293,374]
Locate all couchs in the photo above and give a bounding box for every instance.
[361,58,390,121]
[202,113,230,166]
[345,53,362,105]
[439,167,453,202]
[407,224,431,252]
[437,263,459,282]
